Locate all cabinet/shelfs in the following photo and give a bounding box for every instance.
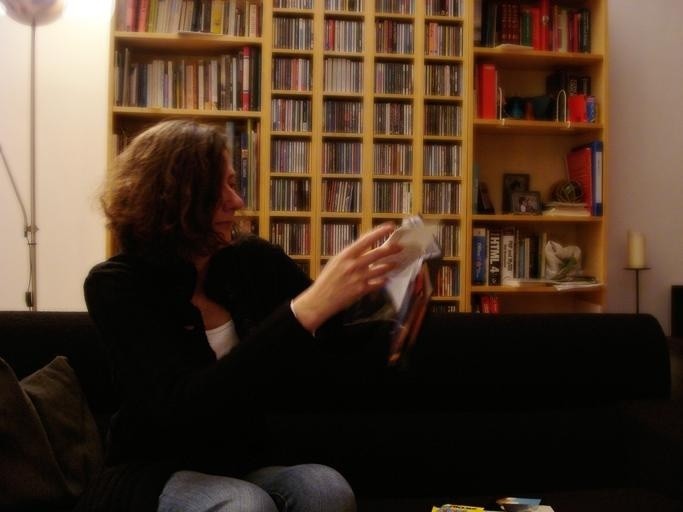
[265,0,463,313]
[107,2,263,235]
[464,0,610,313]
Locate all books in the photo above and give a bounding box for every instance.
[115,1,260,210]
[473,61,592,123]
[270,1,463,313]
[470,224,556,287]
[542,141,603,216]
[472,2,593,54]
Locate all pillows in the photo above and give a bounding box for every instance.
[2,355,108,505]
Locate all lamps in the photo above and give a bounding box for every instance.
[622,229,651,313]
[1,0,64,311]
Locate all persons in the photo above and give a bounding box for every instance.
[84,119,403,512]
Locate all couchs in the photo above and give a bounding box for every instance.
[0,311,683,512]
